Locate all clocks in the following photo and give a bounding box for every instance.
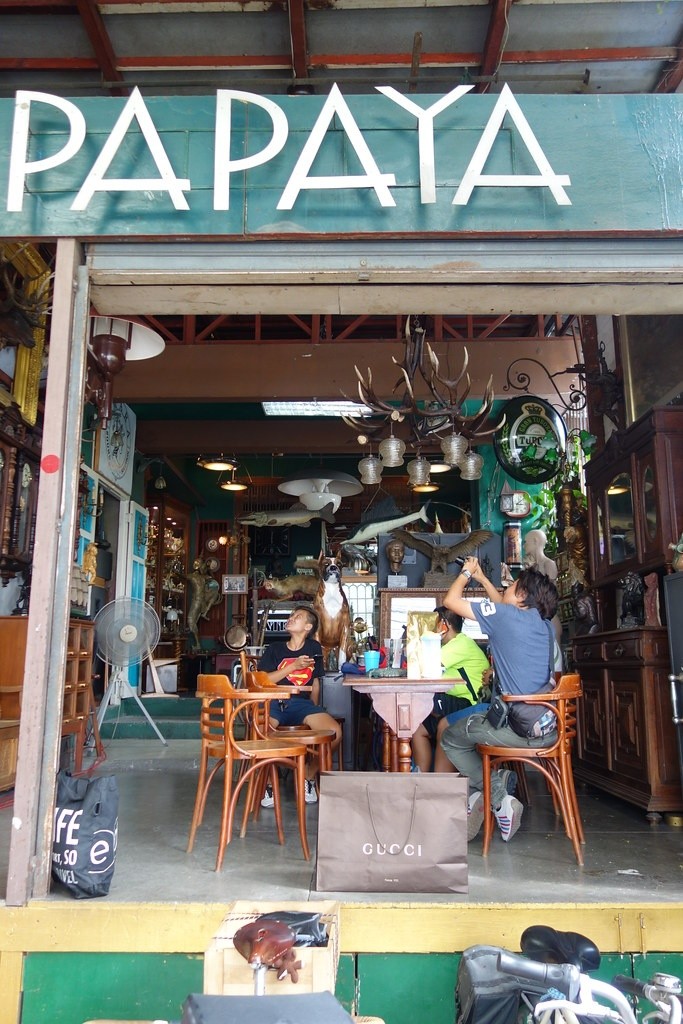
[506,490,530,517]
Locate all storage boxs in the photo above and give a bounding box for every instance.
[204,902,340,995]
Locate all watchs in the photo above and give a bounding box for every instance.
[460,570,472,580]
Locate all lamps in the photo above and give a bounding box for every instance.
[197,453,238,471]
[83,316,167,432]
[137,455,166,489]
[277,466,363,514]
[217,457,255,491]
[339,313,508,485]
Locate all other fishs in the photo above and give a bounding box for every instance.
[236,502,336,528]
[340,499,435,545]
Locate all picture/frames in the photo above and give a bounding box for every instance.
[0,243,51,424]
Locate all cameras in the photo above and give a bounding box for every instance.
[455,556,468,567]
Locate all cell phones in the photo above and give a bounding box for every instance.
[311,654,324,661]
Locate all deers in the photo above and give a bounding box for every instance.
[0,242,57,351]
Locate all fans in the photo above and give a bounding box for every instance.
[90,596,167,746]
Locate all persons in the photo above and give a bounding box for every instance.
[522,531,558,581]
[410,556,564,843]
[171,558,219,648]
[257,606,342,808]
[387,538,406,573]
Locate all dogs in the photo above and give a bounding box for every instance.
[311,549,354,674]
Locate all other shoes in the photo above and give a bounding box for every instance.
[498,769,518,795]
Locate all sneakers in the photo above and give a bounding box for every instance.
[494,795,524,843]
[261,785,274,808]
[305,780,317,804]
[467,791,484,842]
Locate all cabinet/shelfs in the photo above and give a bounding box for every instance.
[1,615,95,795]
[142,493,195,692]
[568,402,683,816]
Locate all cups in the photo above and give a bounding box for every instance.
[364,652,380,675]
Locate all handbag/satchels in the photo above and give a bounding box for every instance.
[508,621,557,745]
[481,696,510,730]
[316,770,469,894]
[52,768,120,899]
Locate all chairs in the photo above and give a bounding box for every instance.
[182,650,344,870]
[478,673,588,867]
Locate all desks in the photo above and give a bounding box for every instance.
[335,671,471,776]
